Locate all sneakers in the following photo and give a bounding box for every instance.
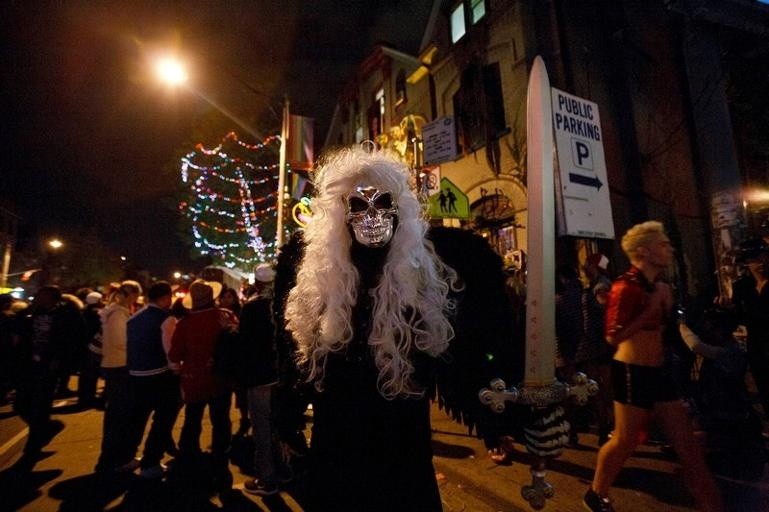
[97,446,299,498]
[581,483,617,512]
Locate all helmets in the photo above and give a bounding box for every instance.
[86,292,103,304]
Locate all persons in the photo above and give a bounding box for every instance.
[1,265,311,494]
[580,253,614,431]
[553,259,611,447]
[271,137,518,511]
[583,217,730,512]
[711,237,769,439]
